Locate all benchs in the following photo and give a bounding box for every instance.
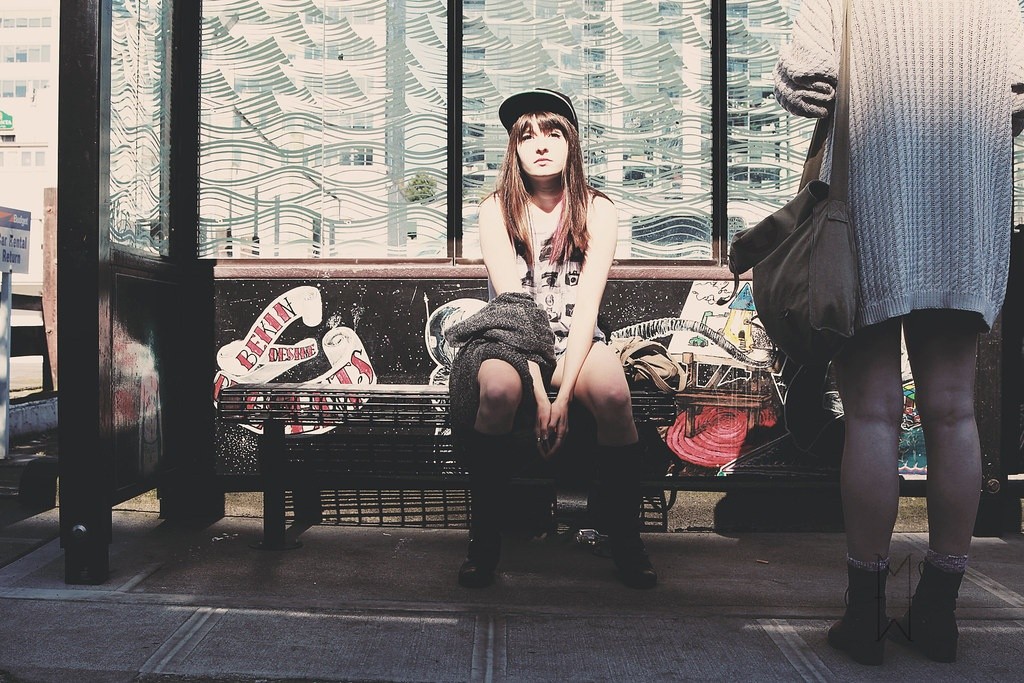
[213,385,775,535]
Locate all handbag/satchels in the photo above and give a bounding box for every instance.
[729,178,859,368]
[611,335,679,391]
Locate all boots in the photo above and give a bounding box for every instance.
[827,561,889,665]
[888,557,965,663]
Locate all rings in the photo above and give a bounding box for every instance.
[537,438,541,442]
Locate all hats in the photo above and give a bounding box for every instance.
[498,87,579,135]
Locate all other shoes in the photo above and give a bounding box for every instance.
[458,523,502,588]
[607,517,657,590]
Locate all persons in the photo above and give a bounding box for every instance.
[448,87,656,590]
[772,0,1024,665]
